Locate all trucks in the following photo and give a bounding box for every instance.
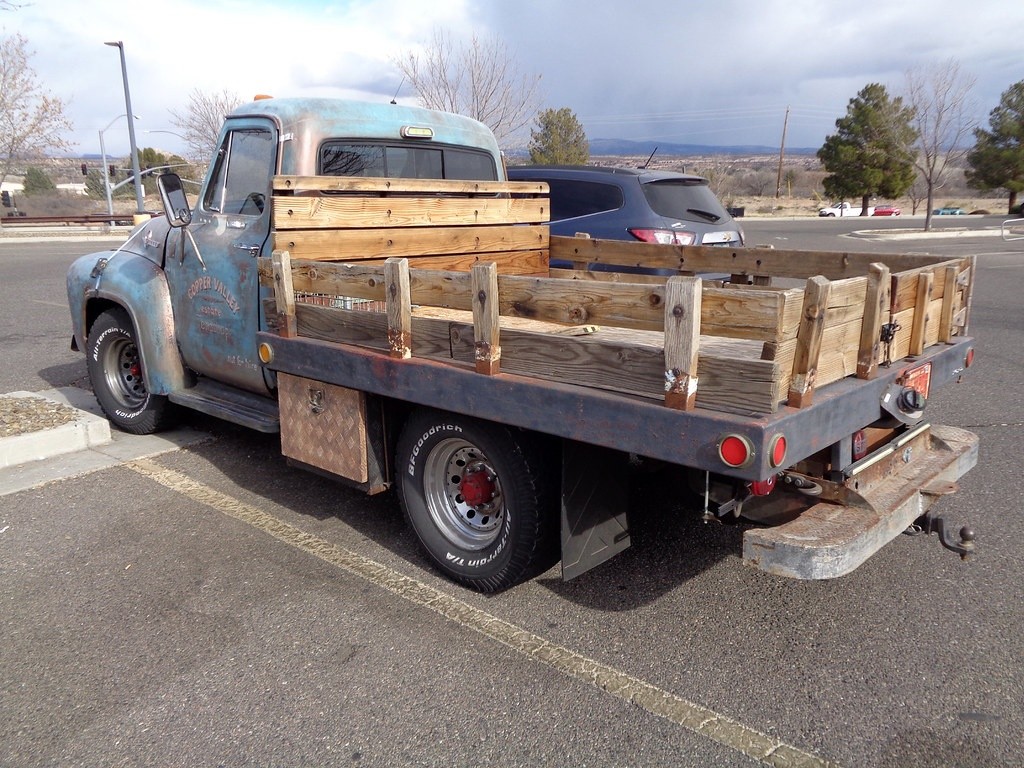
[66,95,981,594]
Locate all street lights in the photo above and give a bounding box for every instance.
[104,42,146,214]
[99,114,141,227]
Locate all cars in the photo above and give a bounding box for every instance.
[933,206,966,216]
[872,204,900,217]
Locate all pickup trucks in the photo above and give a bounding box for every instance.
[819,201,876,217]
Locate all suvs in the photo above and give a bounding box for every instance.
[507,164,753,285]
